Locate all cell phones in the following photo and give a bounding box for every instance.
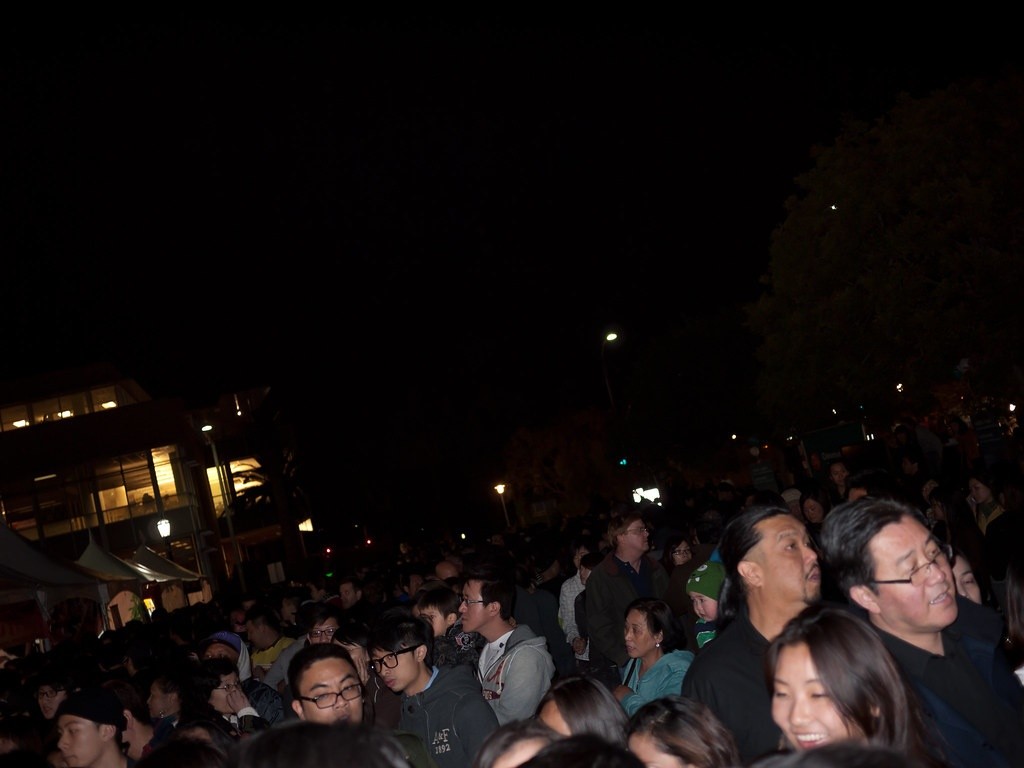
[971,497,975,502]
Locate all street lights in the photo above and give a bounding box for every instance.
[200,423,249,595]
[600,328,631,463]
[494,482,512,530]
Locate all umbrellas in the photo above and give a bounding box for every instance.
[131,535,210,594]
[73,522,180,598]
[1,521,139,603]
[116,543,134,561]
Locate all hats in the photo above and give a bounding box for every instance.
[57,686,128,731]
[206,632,242,653]
[781,488,801,503]
[686,560,727,600]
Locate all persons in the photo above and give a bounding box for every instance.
[142,493,156,513]
[0,552,646,768]
[162,494,178,507]
[216,501,224,516]
[396,359,1024,768]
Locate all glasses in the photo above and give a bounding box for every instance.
[300,683,360,709]
[369,646,418,673]
[459,596,486,605]
[875,544,952,587]
[627,527,647,535]
[674,549,690,555]
[310,628,337,638]
[215,682,240,692]
[39,689,63,697]
